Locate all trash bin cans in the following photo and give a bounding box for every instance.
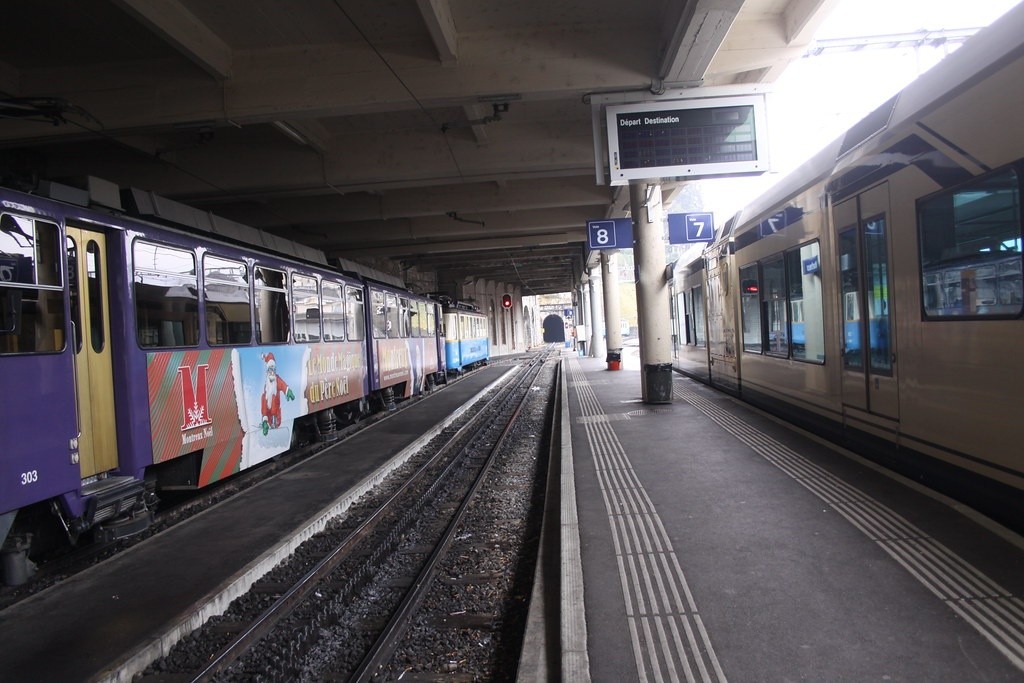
[608,349,623,370]
[644,362,674,404]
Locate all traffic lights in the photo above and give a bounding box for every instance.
[502,293,512,309]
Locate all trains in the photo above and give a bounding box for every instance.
[0,188,495,590]
[670,0,1024,508]
